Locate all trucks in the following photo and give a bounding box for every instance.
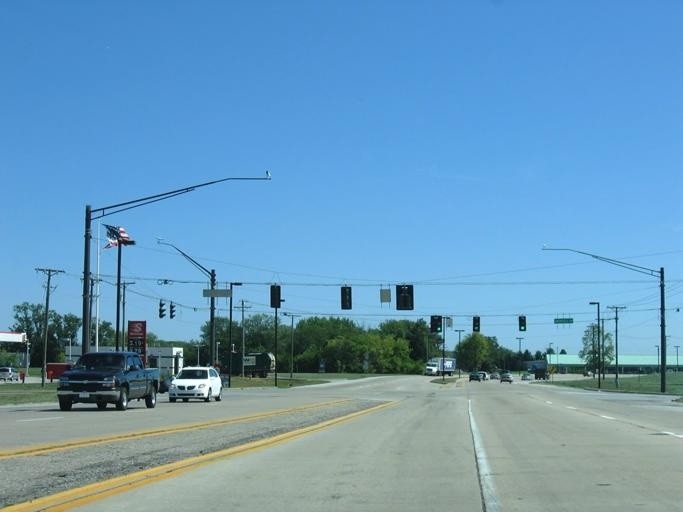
[425,357,456,376]
[533,361,550,380]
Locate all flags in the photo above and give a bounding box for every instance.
[102,222,129,249]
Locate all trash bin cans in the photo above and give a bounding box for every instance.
[221,374,229,387]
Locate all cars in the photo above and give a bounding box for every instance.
[167,366,223,402]
[0,366,20,382]
[522,373,532,380]
[469,370,489,381]
[490,370,513,383]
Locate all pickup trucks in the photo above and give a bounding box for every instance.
[57,351,159,411]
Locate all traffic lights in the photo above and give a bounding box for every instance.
[431,315,442,334]
[158,301,166,318]
[169,304,176,318]
[518,315,526,332]
[472,317,481,332]
